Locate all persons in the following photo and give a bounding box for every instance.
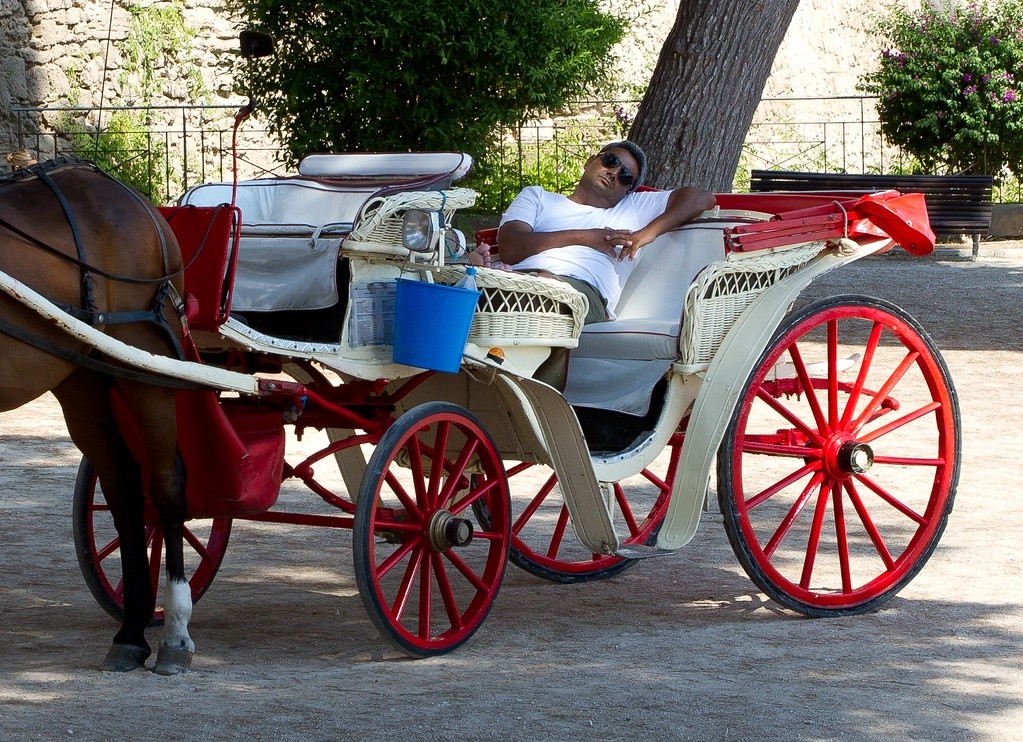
[468,140,717,325]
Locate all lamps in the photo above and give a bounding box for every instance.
[402,206,441,255]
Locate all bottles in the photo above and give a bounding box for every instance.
[452,267,478,291]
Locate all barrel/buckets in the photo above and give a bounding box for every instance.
[392,233,482,373]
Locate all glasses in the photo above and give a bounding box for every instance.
[598,152,633,190]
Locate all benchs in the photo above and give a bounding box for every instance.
[749,169,994,263]
[493,197,807,367]
[175,149,473,316]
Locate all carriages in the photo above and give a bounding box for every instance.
[0,150,962,676]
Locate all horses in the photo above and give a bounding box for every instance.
[0,167,196,676]
